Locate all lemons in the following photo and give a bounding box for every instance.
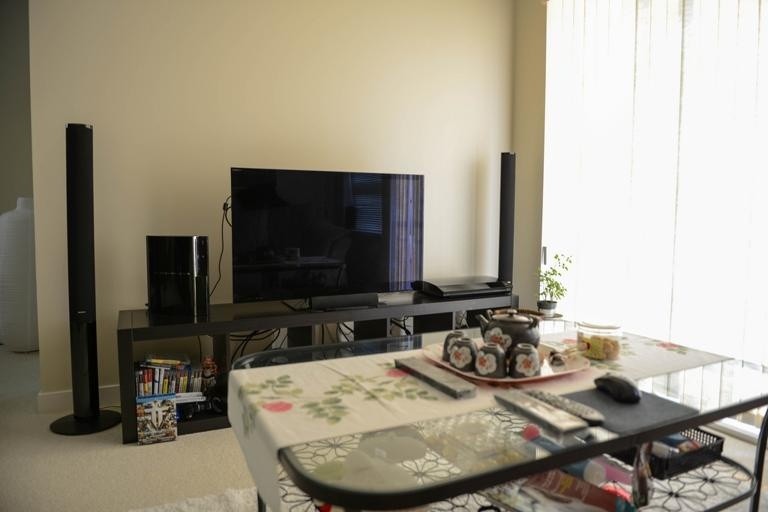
[475,309,540,348]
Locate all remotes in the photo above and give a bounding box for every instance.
[594,372,642,404]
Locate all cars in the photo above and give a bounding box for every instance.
[441,330,465,361]
[509,342,542,376]
[450,338,476,371]
[474,342,505,376]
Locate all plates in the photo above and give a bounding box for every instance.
[117,291,520,445]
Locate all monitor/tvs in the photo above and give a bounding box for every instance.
[135,357,202,445]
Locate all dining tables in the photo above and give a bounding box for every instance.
[532,251,572,314]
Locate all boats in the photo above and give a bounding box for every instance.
[49,124,122,436]
[499,152,515,284]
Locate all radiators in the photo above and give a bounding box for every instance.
[524,388,605,426]
[395,358,477,400]
[494,390,589,438]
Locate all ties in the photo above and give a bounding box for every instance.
[230,167,424,313]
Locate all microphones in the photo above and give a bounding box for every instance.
[539,312,566,333]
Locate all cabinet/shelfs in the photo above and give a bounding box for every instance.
[201,355,218,389]
[575,317,620,361]
